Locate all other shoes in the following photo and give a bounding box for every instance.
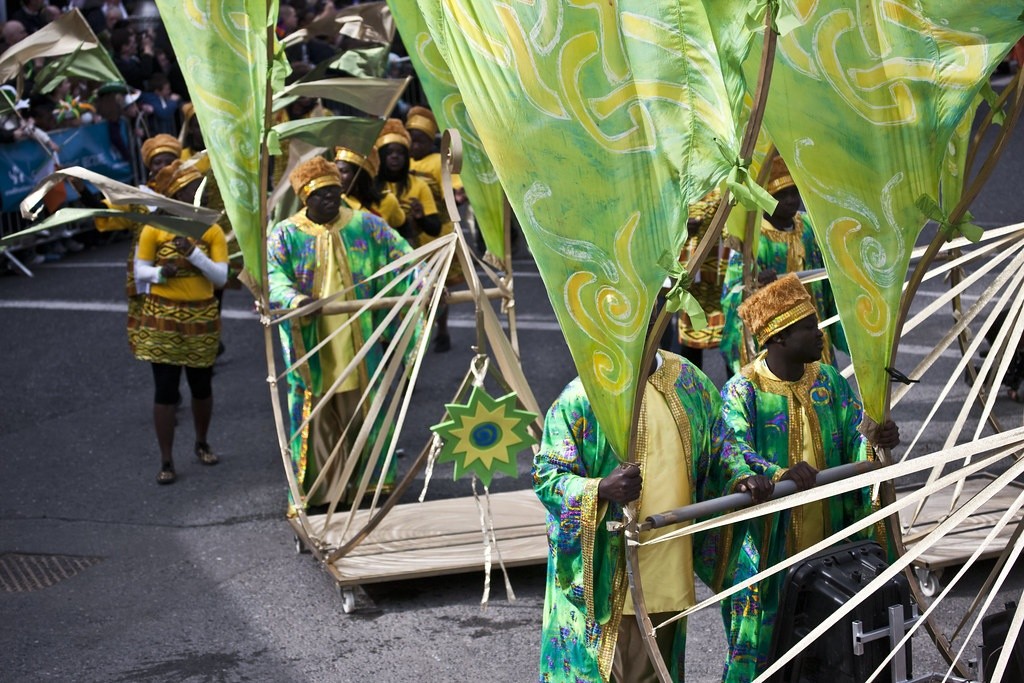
[193,444,219,465]
[157,465,175,484]
[434,334,449,351]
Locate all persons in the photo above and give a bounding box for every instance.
[0,0,466,516]
[533,158,900,683]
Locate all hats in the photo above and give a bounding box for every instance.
[334,142,380,179]
[140,134,181,166]
[767,155,796,196]
[154,159,202,197]
[736,272,816,346]
[405,105,439,142]
[375,118,412,149]
[290,156,341,206]
